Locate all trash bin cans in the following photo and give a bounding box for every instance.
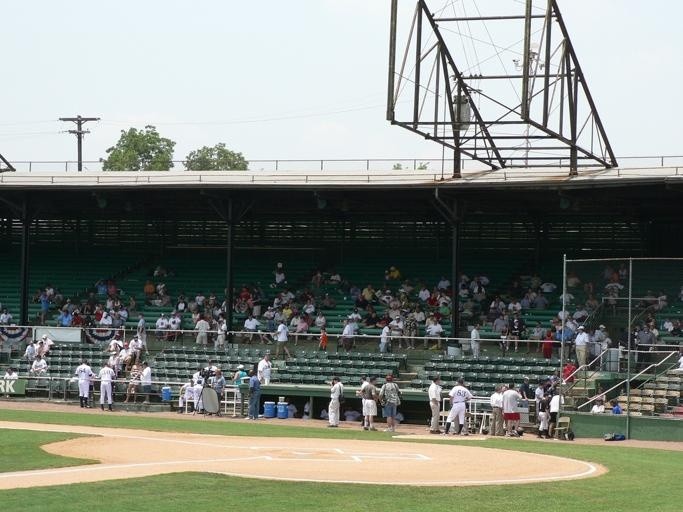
[606,348,619,372]
[517,399,530,424]
[448,342,462,356]
[264,401,289,418]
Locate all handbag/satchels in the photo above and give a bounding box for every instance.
[339,394,345,404]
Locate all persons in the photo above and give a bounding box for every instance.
[303,398,310,419]
[457,263,627,370]
[25,335,55,382]
[490,372,564,439]
[633,292,682,373]
[4,368,18,398]
[584,388,622,414]
[143,264,173,308]
[338,267,452,354]
[321,406,330,420]
[1,308,13,325]
[344,406,361,421]
[234,262,343,351]
[232,353,272,419]
[428,377,472,435]
[272,322,291,360]
[137,293,227,355]
[75,335,152,411]
[562,359,576,396]
[287,399,297,418]
[330,377,344,426]
[178,361,225,415]
[36,279,136,328]
[356,376,404,432]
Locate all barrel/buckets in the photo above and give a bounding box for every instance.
[276,402,288,419]
[263,401,276,418]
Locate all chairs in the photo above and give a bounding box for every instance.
[0,342,682,419]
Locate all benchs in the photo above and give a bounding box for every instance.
[1,239,680,342]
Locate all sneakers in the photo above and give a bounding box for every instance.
[383,428,394,431]
[364,426,377,430]
[508,431,520,437]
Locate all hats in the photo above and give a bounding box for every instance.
[237,365,244,368]
[103,312,107,317]
[578,326,584,329]
[134,335,138,340]
[124,343,128,347]
[599,325,606,328]
[117,333,121,337]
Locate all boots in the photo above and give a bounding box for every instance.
[538,429,551,438]
[80,397,90,408]
[444,423,450,434]
[101,404,112,412]
[457,424,463,434]
